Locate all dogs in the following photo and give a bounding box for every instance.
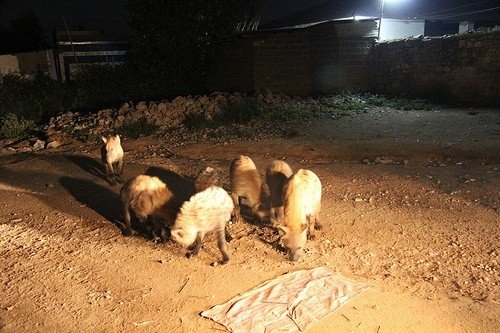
[101,134,323,265]
